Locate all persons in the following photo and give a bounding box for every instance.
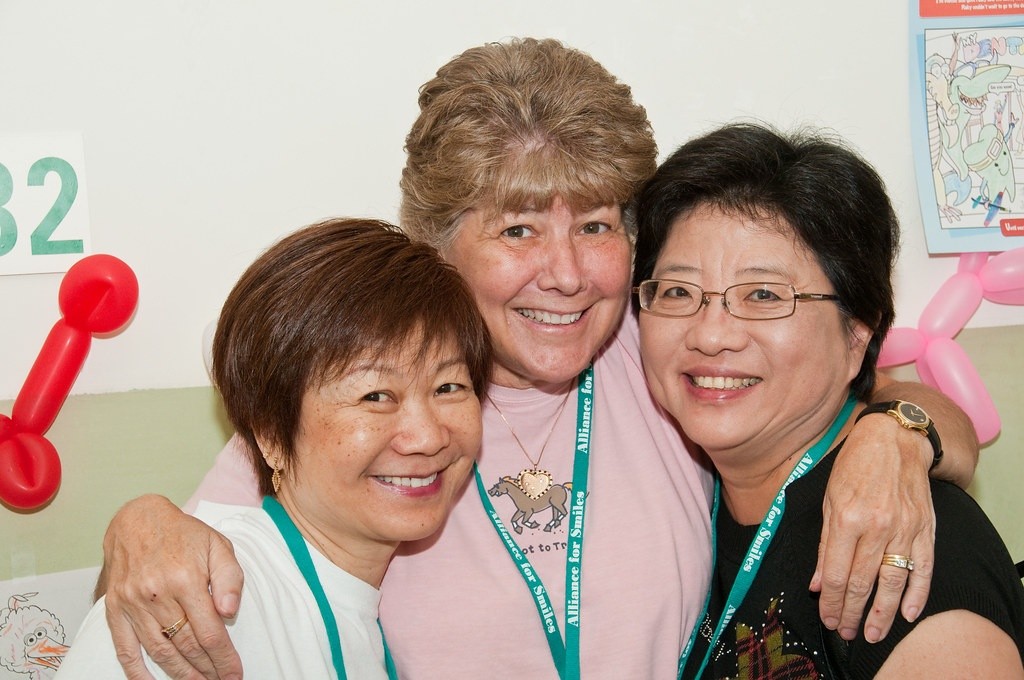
[93,38,980,680]
[631,123,1024,680]
[53,220,494,680]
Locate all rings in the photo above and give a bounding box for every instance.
[881,555,914,571]
[161,615,188,639]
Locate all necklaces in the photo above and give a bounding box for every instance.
[484,377,577,501]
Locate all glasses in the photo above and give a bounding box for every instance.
[633,280,842,321]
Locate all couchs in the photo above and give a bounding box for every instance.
[2,325,1023,680]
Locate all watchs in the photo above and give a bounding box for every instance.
[854,398,944,473]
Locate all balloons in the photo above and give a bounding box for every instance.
[0,254,139,511]
[876,247,1024,445]
